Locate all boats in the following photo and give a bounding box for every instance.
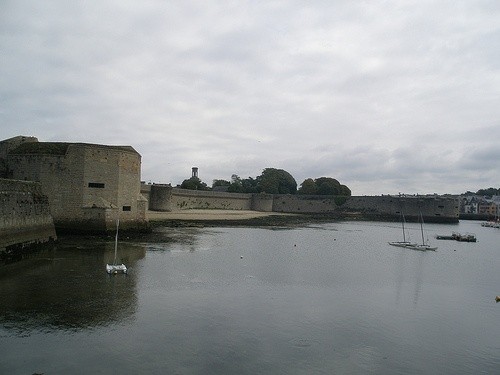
[434,232,477,243]
[481,220,500,228]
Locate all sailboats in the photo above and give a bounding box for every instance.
[105,218,127,275]
[404,211,438,252]
[385,210,417,248]
